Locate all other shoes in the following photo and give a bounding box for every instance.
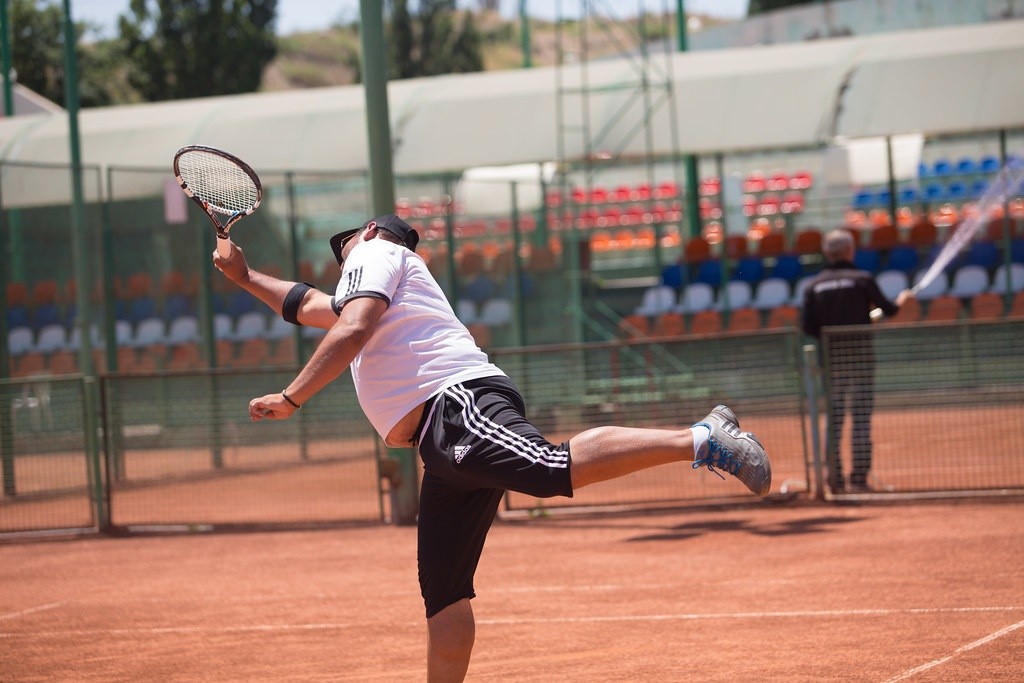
[830,484,844,496]
[850,480,873,494]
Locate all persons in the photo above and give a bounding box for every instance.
[213,213,772,682]
[799,229,917,493]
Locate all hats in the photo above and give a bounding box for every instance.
[818,229,854,256]
[330,214,420,265]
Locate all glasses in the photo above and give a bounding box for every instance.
[341,233,357,250]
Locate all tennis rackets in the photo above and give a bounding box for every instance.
[171,145,264,259]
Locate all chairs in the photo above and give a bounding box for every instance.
[0,157,1023,374]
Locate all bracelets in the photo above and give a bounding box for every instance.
[282,390,300,408]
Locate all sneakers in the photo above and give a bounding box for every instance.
[689,404,772,498]
